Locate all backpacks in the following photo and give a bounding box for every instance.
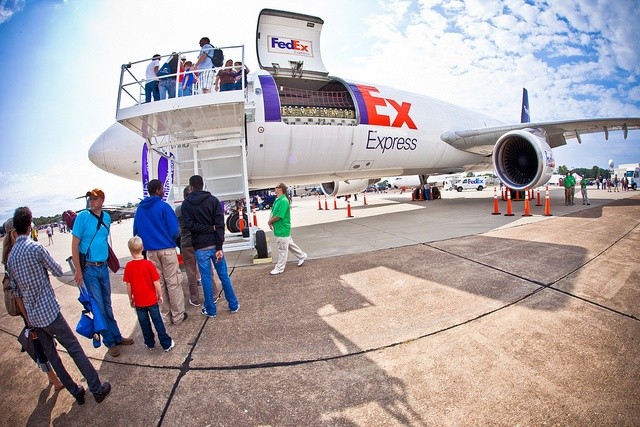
[202,43,224,67]
[156,54,171,75]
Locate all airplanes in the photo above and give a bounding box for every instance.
[388,175,465,190]
[75,192,136,224]
[304,186,324,195]
[88,8,640,252]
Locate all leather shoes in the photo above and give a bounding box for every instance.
[116,337,134,344]
[75,384,85,406]
[93,381,112,403]
[107,345,120,357]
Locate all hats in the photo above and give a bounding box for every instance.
[3,218,17,232]
[85,188,105,200]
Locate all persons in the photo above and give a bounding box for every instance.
[182,61,194,96]
[580,175,590,205]
[189,36,217,94]
[32,226,38,241]
[72,188,134,357]
[4,206,112,405]
[158,52,178,100]
[563,172,570,206]
[215,59,235,92]
[570,171,576,205]
[133,179,188,326]
[227,61,250,90]
[123,235,175,352]
[145,53,160,102]
[1,218,64,392]
[180,175,240,318]
[45,224,54,245]
[267,183,307,274]
[178,56,187,97]
[596,174,630,193]
[50,224,54,235]
[175,186,221,307]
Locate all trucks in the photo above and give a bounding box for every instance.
[626,168,640,190]
[432,179,453,192]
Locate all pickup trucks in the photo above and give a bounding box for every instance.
[454,179,487,192]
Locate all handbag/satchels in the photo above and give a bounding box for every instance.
[157,63,171,77]
[78,210,104,273]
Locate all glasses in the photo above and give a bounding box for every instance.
[89,197,98,200]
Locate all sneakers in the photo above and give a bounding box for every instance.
[200,308,216,318]
[231,303,240,313]
[213,294,221,304]
[162,339,175,352]
[188,298,201,307]
[297,253,308,266]
[145,344,156,350]
[269,267,284,274]
[173,312,188,325]
[197,279,202,286]
